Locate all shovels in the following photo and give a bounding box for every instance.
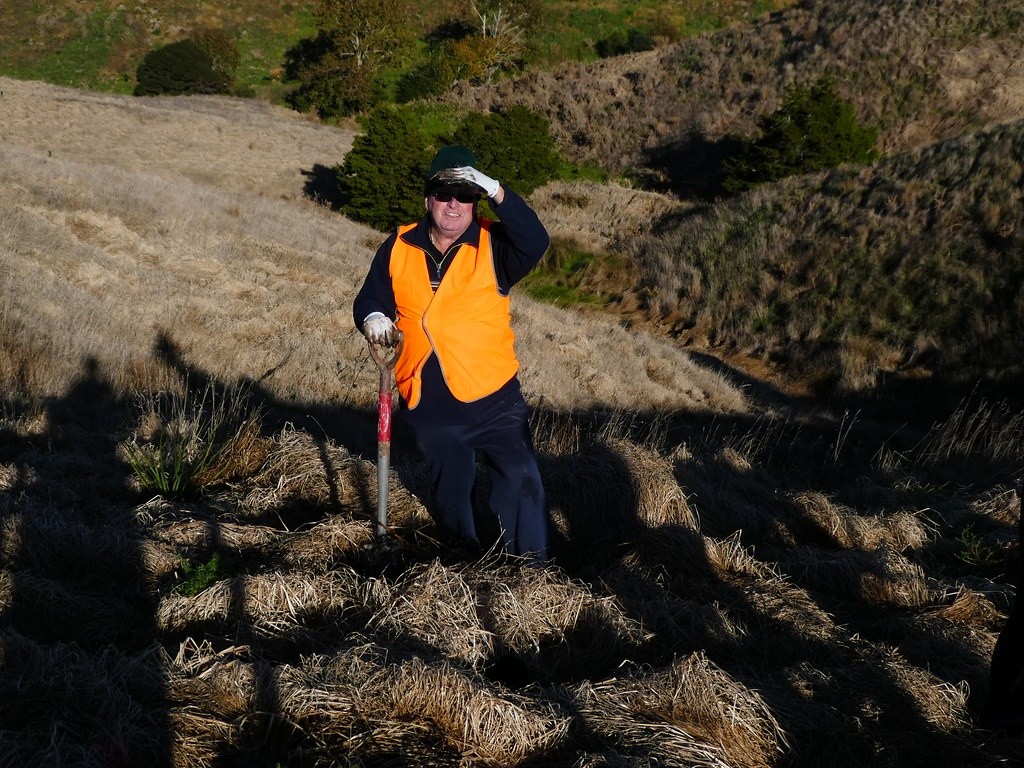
[363,330,404,577]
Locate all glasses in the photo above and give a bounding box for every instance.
[429,190,481,203]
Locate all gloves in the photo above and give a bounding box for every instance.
[363,313,398,348]
[441,166,499,198]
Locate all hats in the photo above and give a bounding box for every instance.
[426,145,476,186]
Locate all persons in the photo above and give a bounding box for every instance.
[353,147,549,568]
